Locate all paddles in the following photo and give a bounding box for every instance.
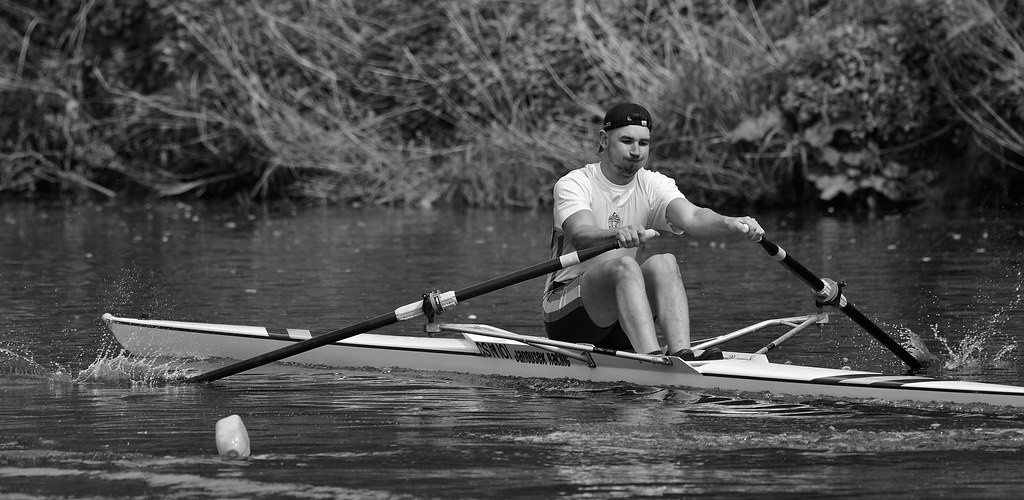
[179,225,658,386]
[758,233,931,372]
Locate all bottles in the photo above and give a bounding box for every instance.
[212,414,253,465]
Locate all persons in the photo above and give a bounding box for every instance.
[541,104,766,364]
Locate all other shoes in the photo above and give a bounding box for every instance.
[670,349,695,361]
[695,346,724,360]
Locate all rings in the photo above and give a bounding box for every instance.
[638,231,643,235]
[753,228,758,231]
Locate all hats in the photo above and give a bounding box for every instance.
[599,103,652,152]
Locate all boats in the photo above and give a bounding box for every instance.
[100,304,1024,417]
